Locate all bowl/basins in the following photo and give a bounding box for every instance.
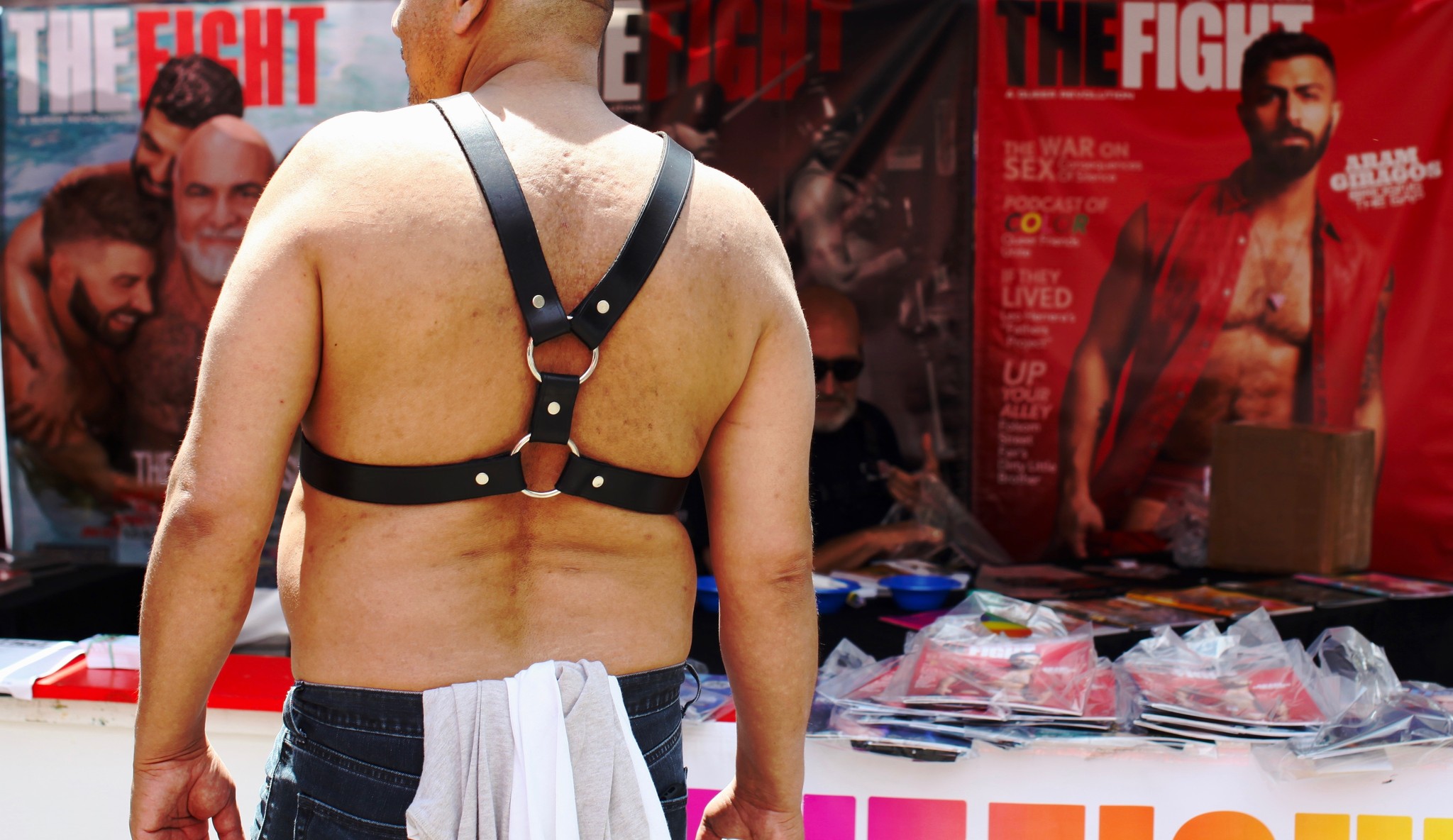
[697,574,862,619]
[876,575,963,612]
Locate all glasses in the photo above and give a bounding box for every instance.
[812,356,868,382]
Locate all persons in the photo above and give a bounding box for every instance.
[797,283,1002,574]
[0,53,305,539]
[124,1,819,840]
[1056,31,1399,566]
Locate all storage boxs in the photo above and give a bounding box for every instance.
[1205,422,1375,574]
